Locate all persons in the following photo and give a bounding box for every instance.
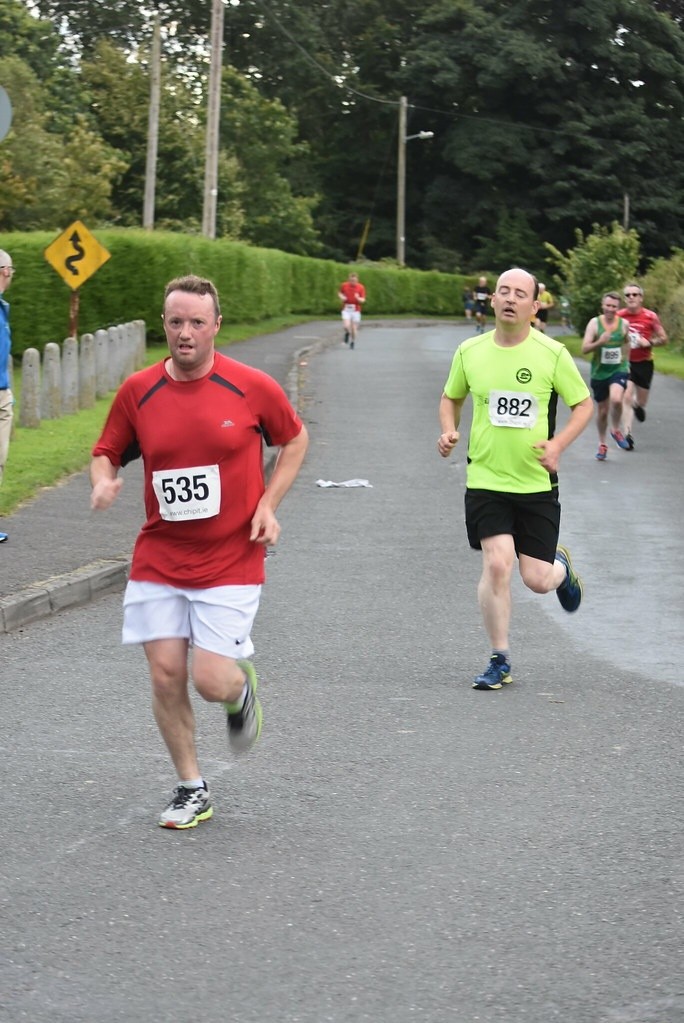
[528,282,573,334]
[438,267,593,690]
[462,276,494,334]
[90,273,309,830]
[337,271,365,350]
[582,291,629,460]
[615,284,667,452]
[0,247,15,543]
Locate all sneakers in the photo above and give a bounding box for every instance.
[471,653,514,690]
[632,402,646,423]
[158,781,214,829]
[625,434,634,451]
[221,659,263,754]
[550,545,584,614]
[610,429,629,449]
[594,443,609,461]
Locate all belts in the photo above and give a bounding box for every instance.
[0,387,8,390]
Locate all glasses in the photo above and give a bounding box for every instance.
[624,293,642,297]
[0,265,17,274]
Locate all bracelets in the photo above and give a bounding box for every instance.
[648,339,654,346]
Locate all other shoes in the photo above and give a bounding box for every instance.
[0,532,8,541]
[343,332,348,342]
[350,342,354,349]
[475,324,480,332]
[481,329,486,334]
